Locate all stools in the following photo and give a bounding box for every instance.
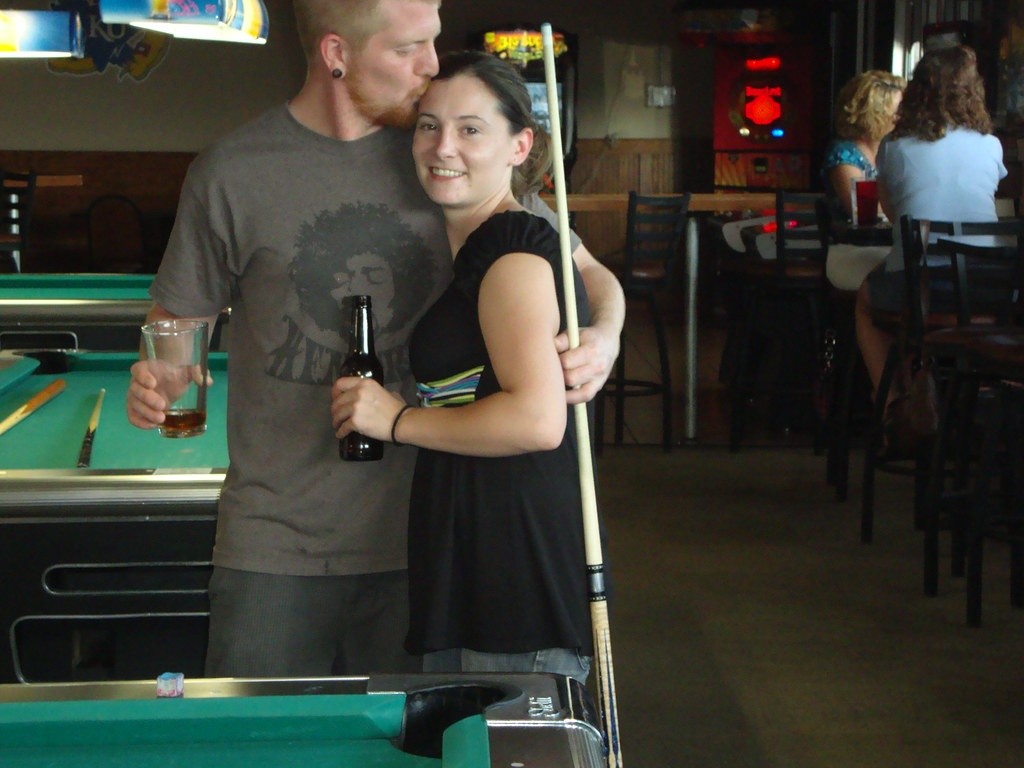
[594,191,691,452]
[864,216,1022,548]
[732,195,840,453]
[922,241,1024,626]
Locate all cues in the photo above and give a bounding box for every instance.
[539,21,622,767]
[77,389,107,469]
[0,378,66,437]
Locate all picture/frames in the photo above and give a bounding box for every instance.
[923,21,968,55]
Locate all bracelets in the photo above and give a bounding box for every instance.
[392,404,416,447]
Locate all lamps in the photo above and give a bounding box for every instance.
[0,9,82,58]
[99,1,269,44]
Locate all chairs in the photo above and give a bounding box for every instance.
[88,194,148,271]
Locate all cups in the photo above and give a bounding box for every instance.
[140,318,209,438]
[851,177,858,227]
[855,180,879,227]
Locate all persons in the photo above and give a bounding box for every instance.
[822,69,910,316]
[855,44,1008,457]
[333,51,598,686]
[126,1,626,679]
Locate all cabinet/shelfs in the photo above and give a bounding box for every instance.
[486,28,576,189]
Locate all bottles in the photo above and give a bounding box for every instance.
[340,295,384,461]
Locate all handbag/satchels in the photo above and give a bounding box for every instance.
[884,355,939,461]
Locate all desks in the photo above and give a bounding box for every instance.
[549,193,774,213]
